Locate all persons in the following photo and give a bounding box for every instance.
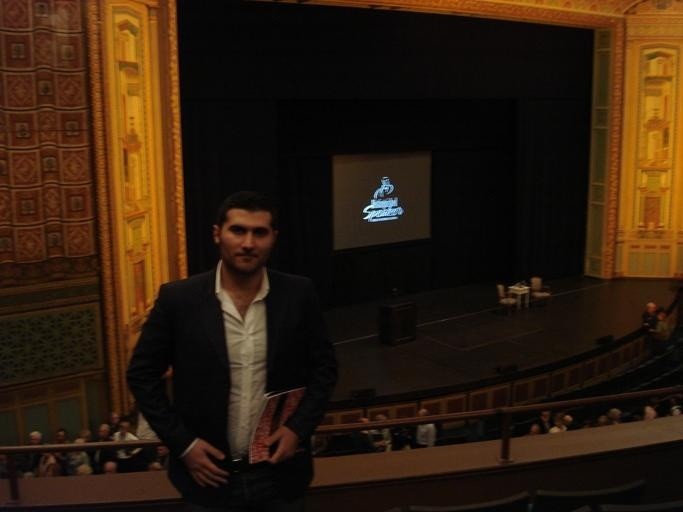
[599,407,682,426]
[2,413,168,478]
[311,407,448,457]
[642,302,670,355]
[530,409,573,434]
[126,190,339,512]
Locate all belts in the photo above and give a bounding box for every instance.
[225,458,249,473]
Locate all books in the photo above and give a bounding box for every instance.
[250,386,306,464]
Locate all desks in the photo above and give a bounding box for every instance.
[508,284,529,312]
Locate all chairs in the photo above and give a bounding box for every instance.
[530,276,554,307]
[497,284,516,317]
